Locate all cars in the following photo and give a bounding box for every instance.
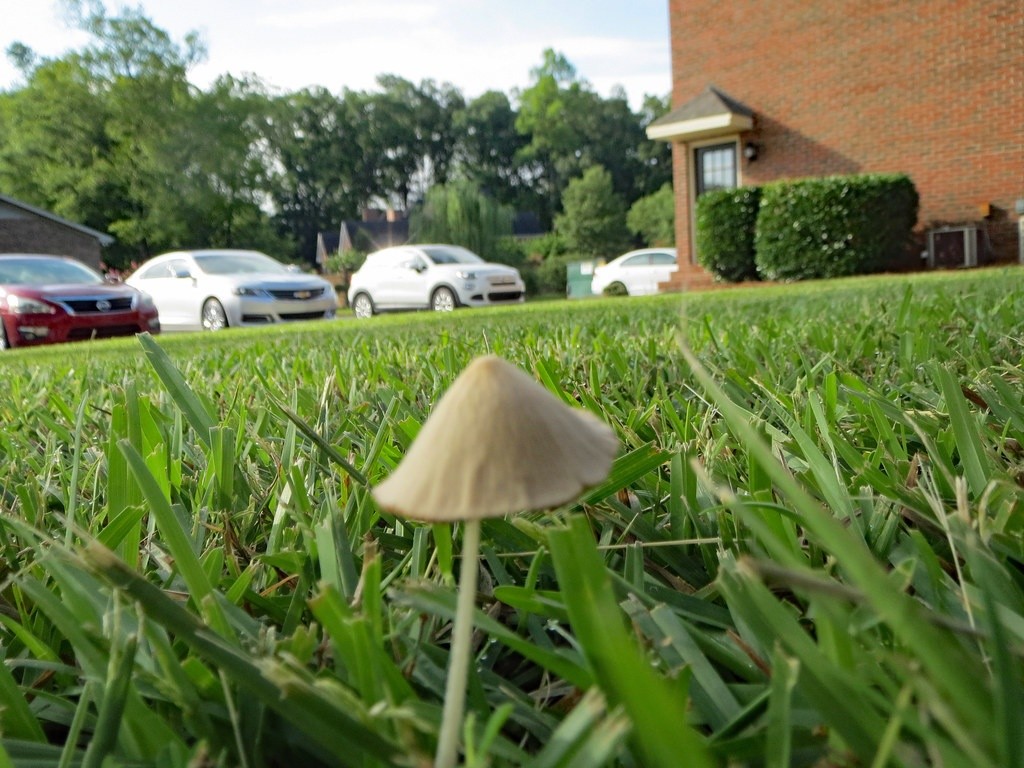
[590,247,679,298]
[346,244,526,319]
[123,248,339,332]
[0,252,161,351]
